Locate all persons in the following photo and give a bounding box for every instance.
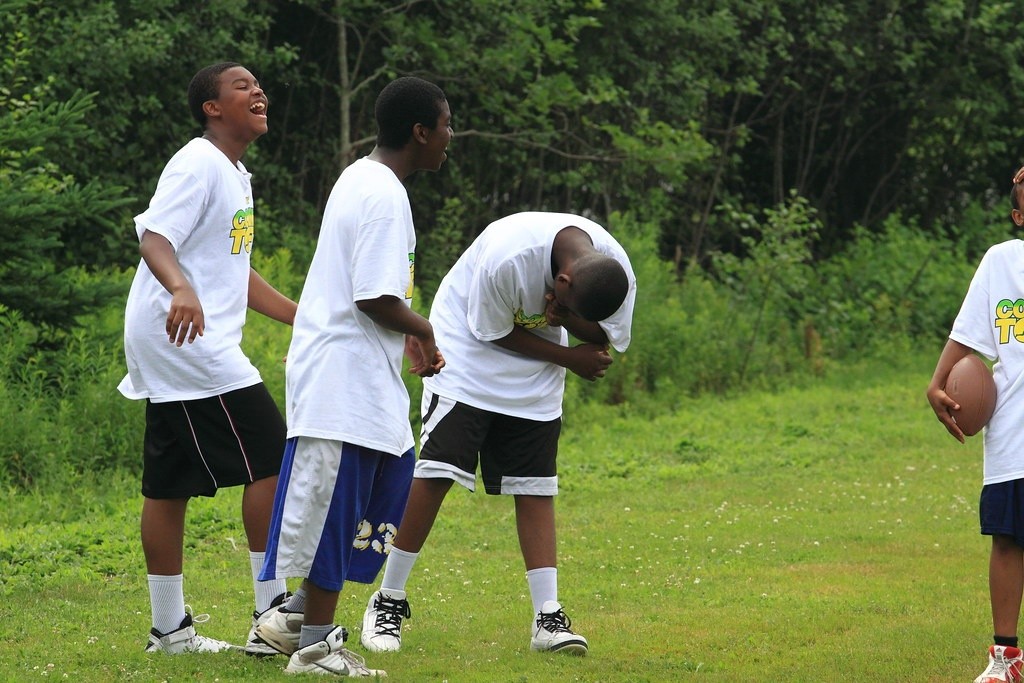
[112,60,327,661]
[360,206,639,661]
[248,74,456,683]
[923,160,1024,683]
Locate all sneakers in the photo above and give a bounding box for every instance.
[529,602,588,654]
[247,590,351,656]
[970,645,1024,683]
[360,586,411,653]
[253,603,302,656]
[142,605,247,657]
[278,628,389,680]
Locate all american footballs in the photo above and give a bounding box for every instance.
[945,354,997,437]
